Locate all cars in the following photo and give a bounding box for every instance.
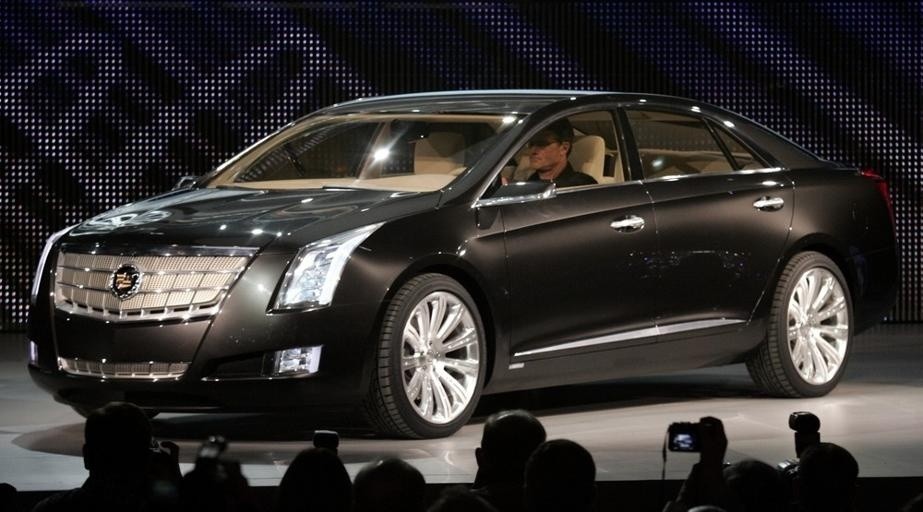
[27,87,901,441]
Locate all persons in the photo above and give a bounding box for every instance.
[0,401,880,507]
[496,114,591,192]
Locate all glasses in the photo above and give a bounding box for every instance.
[529,137,569,147]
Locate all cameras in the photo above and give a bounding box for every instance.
[673,420,704,453]
